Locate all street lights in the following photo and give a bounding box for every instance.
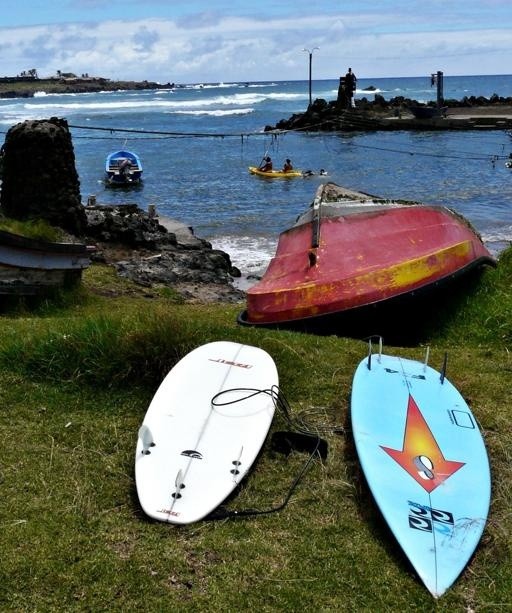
[301,46,320,103]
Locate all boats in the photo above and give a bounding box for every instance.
[106,150,142,185]
[250,166,303,178]
[237,183,489,326]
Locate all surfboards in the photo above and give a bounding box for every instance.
[351,337,492,600]
[134,339,279,524]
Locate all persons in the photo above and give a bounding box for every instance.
[257,156,272,172]
[282,158,292,172]
